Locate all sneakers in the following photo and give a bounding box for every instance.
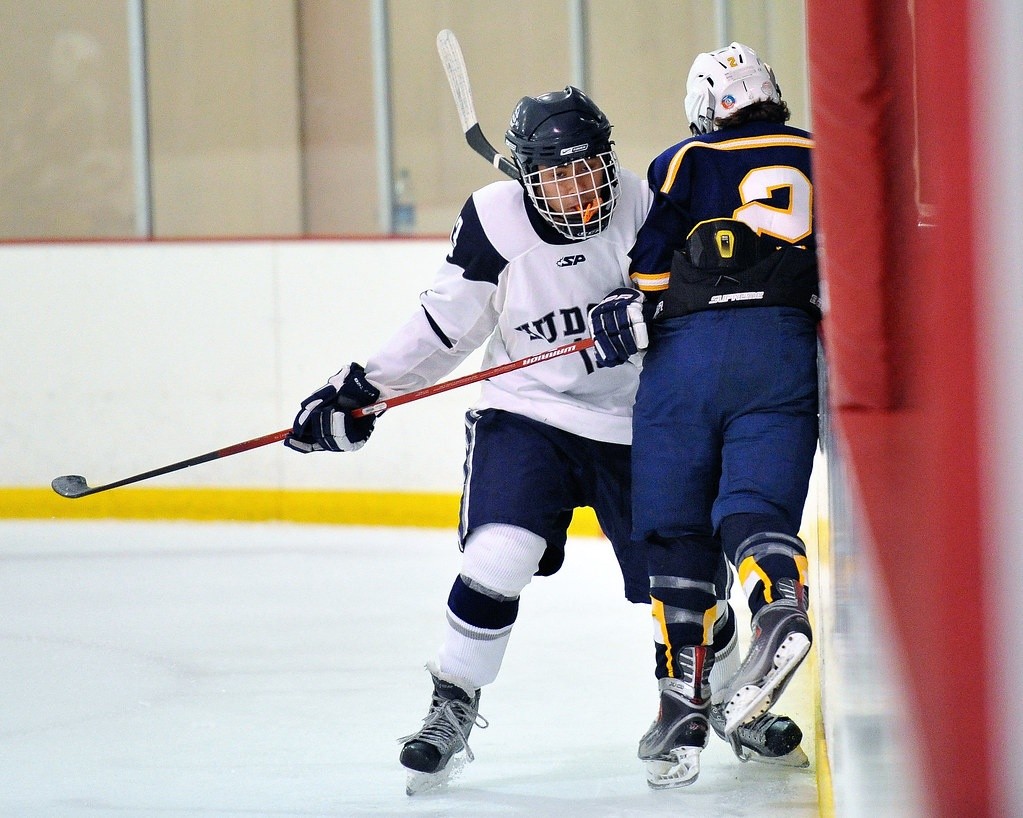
[710,694,810,767]
[718,576,812,737]
[638,647,711,789]
[394,669,488,797]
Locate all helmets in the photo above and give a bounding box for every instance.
[685,42,781,136]
[504,84,622,244]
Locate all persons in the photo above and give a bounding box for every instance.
[632,45,823,783]
[286,88,808,794]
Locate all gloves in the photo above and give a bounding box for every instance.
[587,285,648,367]
[283,362,385,454]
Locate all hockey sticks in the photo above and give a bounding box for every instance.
[436,29,521,181]
[50,337,595,500]
